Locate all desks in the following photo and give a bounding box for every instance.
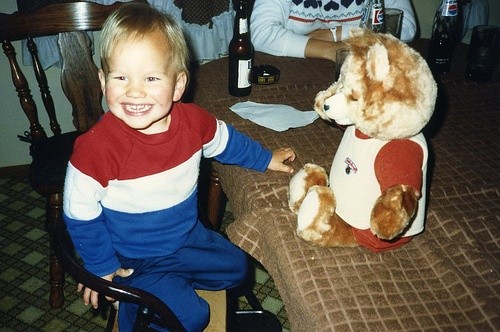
[192,34,500,332]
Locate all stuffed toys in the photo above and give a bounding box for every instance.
[288,28,437,251]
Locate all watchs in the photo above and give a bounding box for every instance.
[330,26,337,43]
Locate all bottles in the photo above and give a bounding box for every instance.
[427,0,458,73]
[229,0,252,96]
[362,0,385,34]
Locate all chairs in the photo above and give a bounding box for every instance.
[0,1,285,332]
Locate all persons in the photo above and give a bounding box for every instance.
[63,2,295,332]
[250,0,416,64]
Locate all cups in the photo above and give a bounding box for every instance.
[383,8,403,39]
[335,47,350,82]
[464,24,500,82]
